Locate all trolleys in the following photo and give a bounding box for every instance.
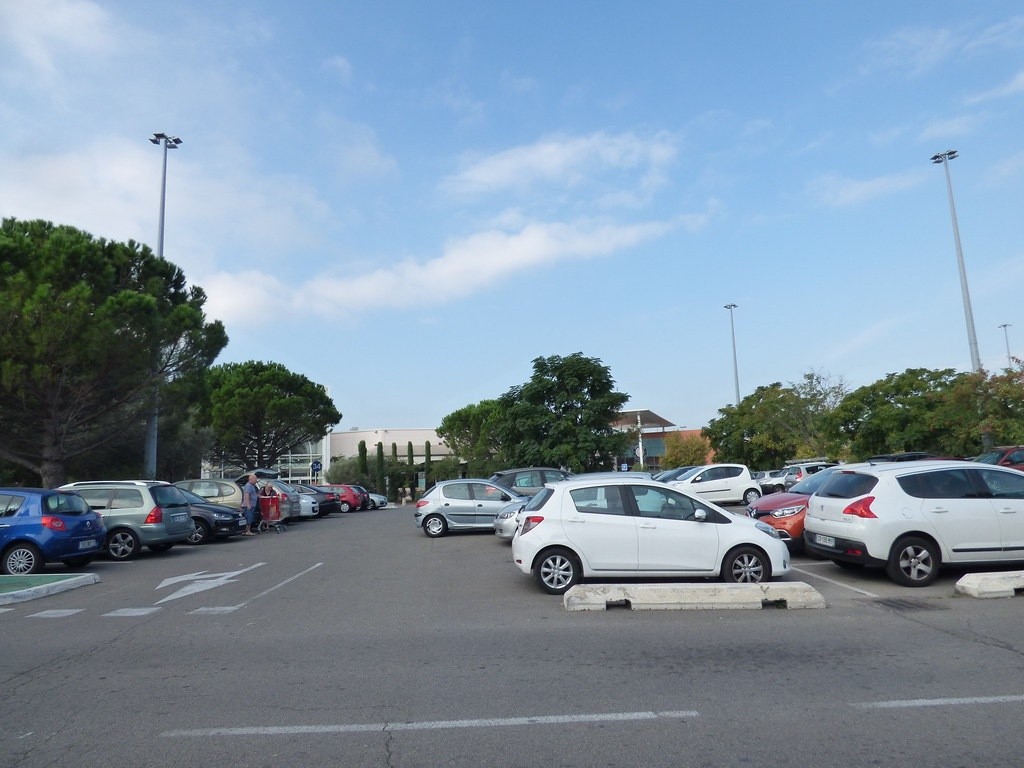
[257,496,290,534]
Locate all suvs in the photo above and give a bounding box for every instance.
[801,459,1023,587]
[246,478,299,521]
[51,480,196,561]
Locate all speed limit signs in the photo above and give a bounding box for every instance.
[311,461,322,472]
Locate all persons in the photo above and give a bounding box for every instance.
[400,487,407,507]
[243,475,258,536]
[257,481,281,533]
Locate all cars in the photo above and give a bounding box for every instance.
[0,486,108,576]
[415,479,534,538]
[490,468,577,500]
[366,493,388,511]
[745,462,903,544]
[786,464,840,492]
[494,472,661,546]
[292,495,319,517]
[287,484,341,519]
[318,485,362,513]
[348,485,370,510]
[511,479,791,598]
[178,486,248,545]
[171,469,279,514]
[975,445,1024,471]
[764,462,826,493]
[662,464,762,505]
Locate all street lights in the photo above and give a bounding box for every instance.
[998,324,1013,369]
[142,130,181,483]
[724,304,740,406]
[930,149,983,373]
